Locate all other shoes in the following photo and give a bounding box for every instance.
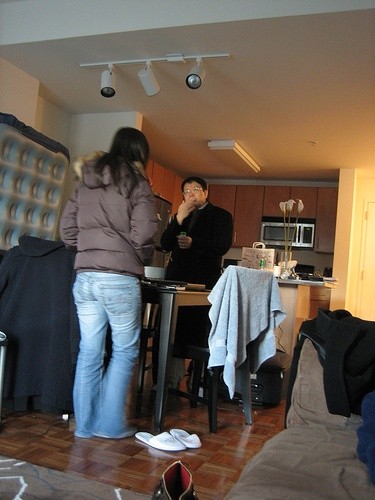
[93,427,137,438]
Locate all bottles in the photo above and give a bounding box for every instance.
[259,259,266,269]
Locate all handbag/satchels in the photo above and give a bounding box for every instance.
[188,354,283,410]
[242,242,275,270]
[152,460,198,500]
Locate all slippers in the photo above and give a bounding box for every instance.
[170,428,202,448]
[135,432,186,451]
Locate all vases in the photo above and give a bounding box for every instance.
[281,252,292,278]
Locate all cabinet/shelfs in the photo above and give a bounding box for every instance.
[146,159,338,254]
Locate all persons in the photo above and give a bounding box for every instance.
[58,126,160,438]
[150,177,236,392]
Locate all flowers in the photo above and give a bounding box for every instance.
[279,199,304,252]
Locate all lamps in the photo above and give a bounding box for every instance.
[207,138,261,176]
[138,60,160,97]
[100,64,116,98]
[185,57,207,90]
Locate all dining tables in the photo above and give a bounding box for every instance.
[137,279,217,434]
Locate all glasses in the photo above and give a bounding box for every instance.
[183,187,205,194]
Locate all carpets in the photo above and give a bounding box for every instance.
[0,455,154,500]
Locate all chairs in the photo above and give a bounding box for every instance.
[167,264,273,432]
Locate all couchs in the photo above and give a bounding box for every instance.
[224,337,375,499]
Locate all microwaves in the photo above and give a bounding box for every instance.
[260,221,316,248]
